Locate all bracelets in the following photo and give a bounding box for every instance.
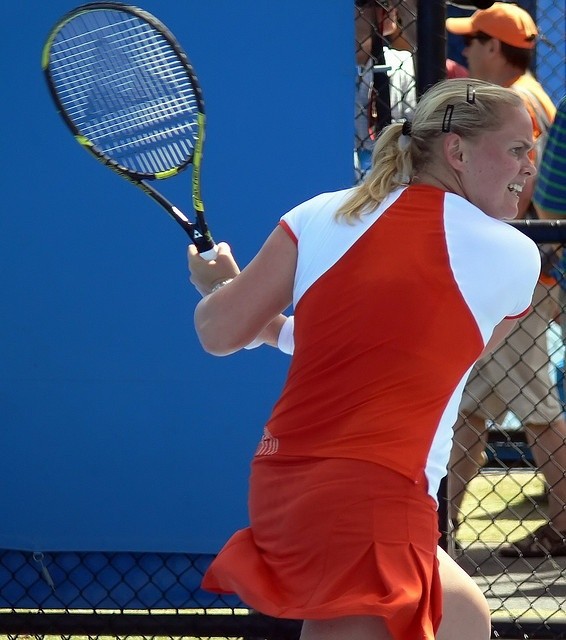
[211,277,234,292]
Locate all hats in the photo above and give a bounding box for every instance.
[446,2,539,50]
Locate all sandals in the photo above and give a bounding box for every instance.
[498,524,566,556]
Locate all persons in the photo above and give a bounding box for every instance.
[532,95,566,367]
[446,1,566,559]
[187,77,543,637]
[353,1,416,185]
[388,1,469,80]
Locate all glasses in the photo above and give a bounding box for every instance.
[461,33,480,47]
